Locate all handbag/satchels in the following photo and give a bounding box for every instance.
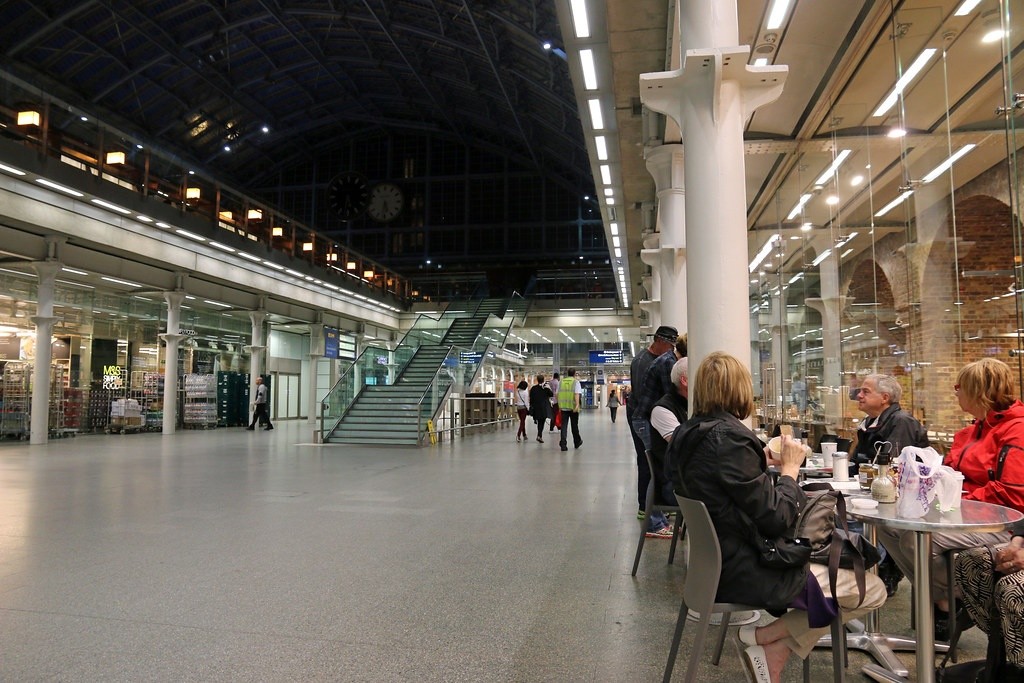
[795,493,882,569]
[752,528,812,571]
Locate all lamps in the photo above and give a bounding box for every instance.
[186,188,200,199]
[247,209,262,219]
[273,227,283,237]
[347,262,356,269]
[106,151,125,166]
[302,243,312,251]
[17,111,40,129]
[364,270,373,277]
[326,253,337,262]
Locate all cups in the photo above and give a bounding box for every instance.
[951,470,966,508]
[820,442,838,468]
[768,437,781,461]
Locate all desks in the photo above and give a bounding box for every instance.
[768,450,1024,683]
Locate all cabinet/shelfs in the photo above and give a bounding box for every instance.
[0,358,219,430]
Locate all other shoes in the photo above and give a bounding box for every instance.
[517,437,521,441]
[737,625,759,647]
[743,645,771,683]
[575,440,583,448]
[549,429,558,434]
[264,424,273,430]
[536,437,544,443]
[246,424,254,430]
[524,437,528,441]
[561,447,567,452]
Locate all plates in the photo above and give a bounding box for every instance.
[851,499,879,508]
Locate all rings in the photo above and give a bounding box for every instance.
[997,548,1003,551]
[1009,562,1014,567]
[1009,568,1015,573]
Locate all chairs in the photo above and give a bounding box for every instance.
[662,489,812,683]
[631,449,687,575]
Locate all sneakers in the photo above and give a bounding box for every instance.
[934,598,977,644]
[640,524,682,539]
[637,510,670,520]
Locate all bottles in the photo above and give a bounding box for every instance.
[859,452,899,504]
[801,431,813,459]
[831,452,849,482]
[753,423,768,443]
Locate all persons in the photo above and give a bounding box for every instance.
[848,371,931,598]
[663,351,886,683]
[554,367,584,451]
[606,390,622,423]
[529,374,554,443]
[649,355,688,505]
[788,371,808,414]
[245,376,274,431]
[626,326,677,520]
[875,357,1024,683]
[516,381,530,442]
[631,332,688,538]
[550,372,562,434]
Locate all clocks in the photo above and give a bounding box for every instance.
[324,171,406,222]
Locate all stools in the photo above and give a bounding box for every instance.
[908,547,971,661]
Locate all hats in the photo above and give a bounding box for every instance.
[646,326,679,347]
